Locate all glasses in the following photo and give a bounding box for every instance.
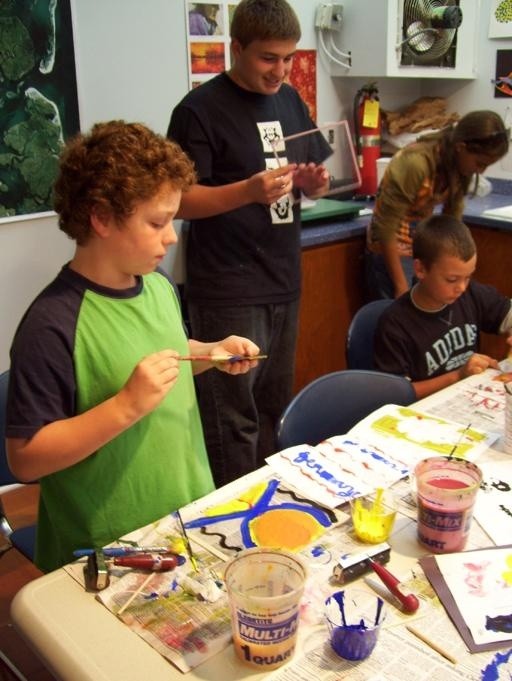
[463,129,511,149]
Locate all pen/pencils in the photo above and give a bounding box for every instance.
[404,624,458,665]
[73,545,170,556]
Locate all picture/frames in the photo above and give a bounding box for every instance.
[0,0,86,228]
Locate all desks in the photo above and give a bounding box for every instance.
[10,356,512,681]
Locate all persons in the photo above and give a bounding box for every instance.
[166,0,333,488]
[366,112,512,298]
[5,119,260,575]
[368,215,512,397]
[189,3,219,35]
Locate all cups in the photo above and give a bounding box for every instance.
[414,456,484,554]
[223,547,310,672]
[321,589,392,662]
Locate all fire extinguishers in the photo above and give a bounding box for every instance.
[350,81,384,200]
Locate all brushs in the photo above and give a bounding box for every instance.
[172,355,242,362]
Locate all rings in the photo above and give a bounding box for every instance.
[280,176,285,189]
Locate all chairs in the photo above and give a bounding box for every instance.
[0,369,40,561]
[276,369,415,454]
[347,298,395,370]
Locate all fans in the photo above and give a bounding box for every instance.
[400,0,463,63]
